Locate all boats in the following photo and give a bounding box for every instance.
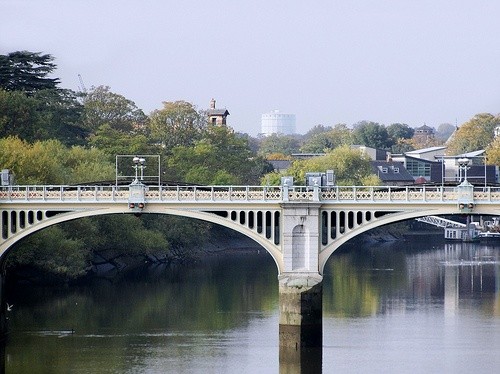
[479,231,500,241]
